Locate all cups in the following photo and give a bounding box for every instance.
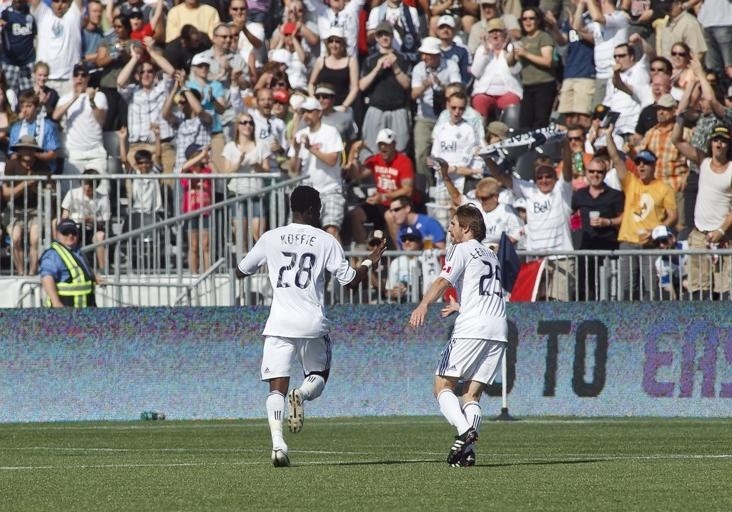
[110,217,124,234]
[589,210,601,227]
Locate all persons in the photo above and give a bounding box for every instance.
[408,204,508,468]
[0,0,731,309]
[235,185,388,467]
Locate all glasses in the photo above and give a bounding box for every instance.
[521,17,534,20]
[672,51,686,57]
[590,169,603,173]
[568,136,581,140]
[614,53,624,57]
[216,7,247,39]
[240,120,254,126]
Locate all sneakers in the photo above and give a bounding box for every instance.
[447,427,478,468]
[271,448,290,467]
[288,388,304,434]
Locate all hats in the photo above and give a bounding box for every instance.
[368,229,385,244]
[652,225,672,242]
[374,21,393,35]
[707,125,731,141]
[375,128,396,144]
[655,93,678,108]
[436,14,455,29]
[185,143,211,157]
[9,135,44,152]
[127,6,142,16]
[481,0,496,6]
[58,219,78,231]
[273,48,291,68]
[487,121,510,139]
[192,53,212,66]
[400,225,421,241]
[74,64,87,72]
[273,87,334,111]
[418,37,442,55]
[324,27,346,39]
[534,155,553,171]
[486,17,505,32]
[634,150,656,162]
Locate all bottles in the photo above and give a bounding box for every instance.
[140,411,165,421]
[574,150,584,173]
